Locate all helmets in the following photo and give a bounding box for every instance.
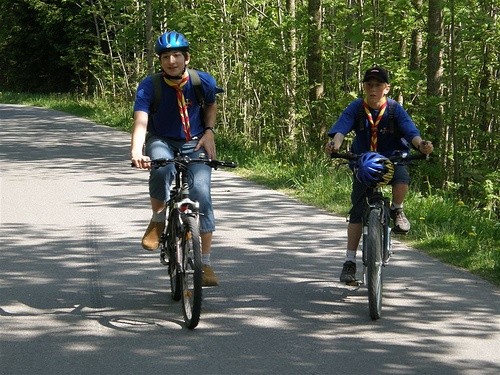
[154,31,189,54]
[354,151,395,187]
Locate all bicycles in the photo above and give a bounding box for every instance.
[129,147,239,330]
[329,140,431,320]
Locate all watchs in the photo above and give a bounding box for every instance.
[203,127,217,134]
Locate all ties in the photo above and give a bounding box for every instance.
[163,70,192,143]
[362,95,387,151]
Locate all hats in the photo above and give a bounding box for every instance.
[363,67,389,83]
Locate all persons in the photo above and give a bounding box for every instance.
[130,32,218,286]
[325,66,434,284]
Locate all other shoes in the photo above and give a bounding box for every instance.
[141,218,166,251]
[389,206,411,232]
[339,260,357,283]
[201,263,220,287]
